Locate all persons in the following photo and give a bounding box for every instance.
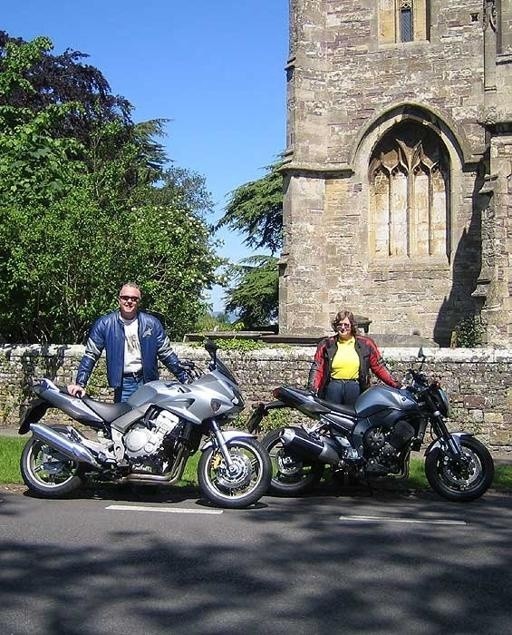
[307,310,406,487]
[67,283,193,502]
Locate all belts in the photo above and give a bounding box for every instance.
[329,377,360,382]
[122,369,144,378]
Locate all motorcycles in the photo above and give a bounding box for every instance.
[17,337,272,508]
[249,345,498,503]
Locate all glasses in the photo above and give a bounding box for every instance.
[119,295,140,302]
[335,323,350,329]
